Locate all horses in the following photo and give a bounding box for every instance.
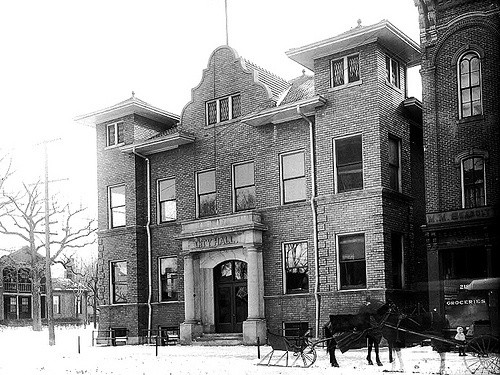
[360,295,453,374]
[322,302,405,368]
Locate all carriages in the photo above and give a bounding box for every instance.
[358,279,500,375]
[254,298,400,368]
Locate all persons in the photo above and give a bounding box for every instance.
[455,326,468,356]
